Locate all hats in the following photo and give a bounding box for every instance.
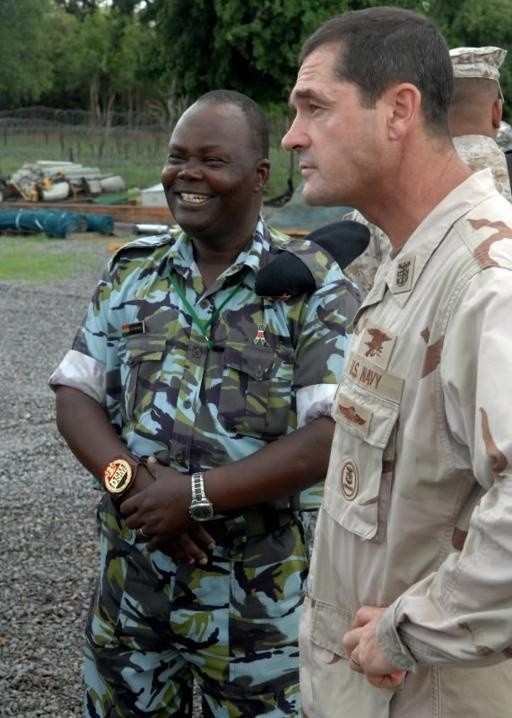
[254,220,370,302]
[449,45,509,104]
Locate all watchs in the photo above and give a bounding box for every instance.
[186,470,217,524]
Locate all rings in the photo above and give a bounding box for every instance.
[139,525,148,539]
[348,650,361,666]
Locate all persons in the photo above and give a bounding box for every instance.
[47,88,365,718]
[444,41,512,204]
[341,207,394,300]
[277,5,512,717]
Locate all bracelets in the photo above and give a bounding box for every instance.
[102,447,146,517]
